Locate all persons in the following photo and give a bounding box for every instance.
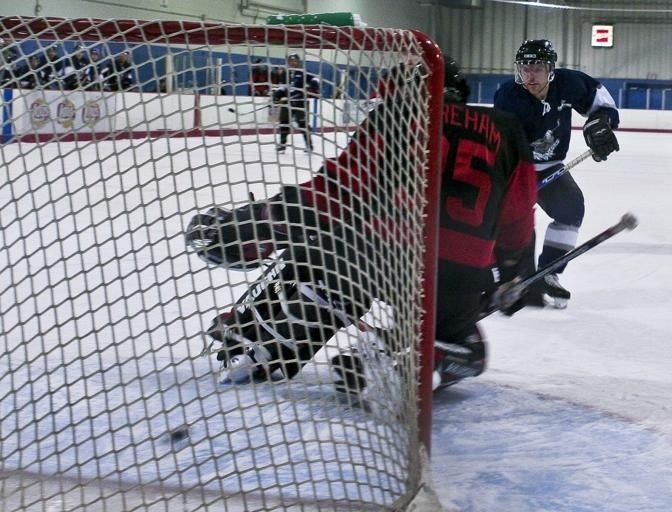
[272,54,320,153]
[0,37,134,92]
[154,79,166,93]
[490,37,618,300]
[184,51,546,403]
[248,57,273,97]
[270,67,288,97]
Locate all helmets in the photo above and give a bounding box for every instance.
[513,39,557,85]
[288,53,299,61]
[381,51,469,101]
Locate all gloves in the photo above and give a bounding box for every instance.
[583,116,619,162]
[311,80,319,90]
[273,90,282,101]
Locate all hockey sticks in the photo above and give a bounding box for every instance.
[299,282,472,359]
[229,95,300,117]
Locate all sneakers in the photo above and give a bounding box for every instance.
[276,146,286,151]
[304,146,312,153]
[535,269,571,298]
